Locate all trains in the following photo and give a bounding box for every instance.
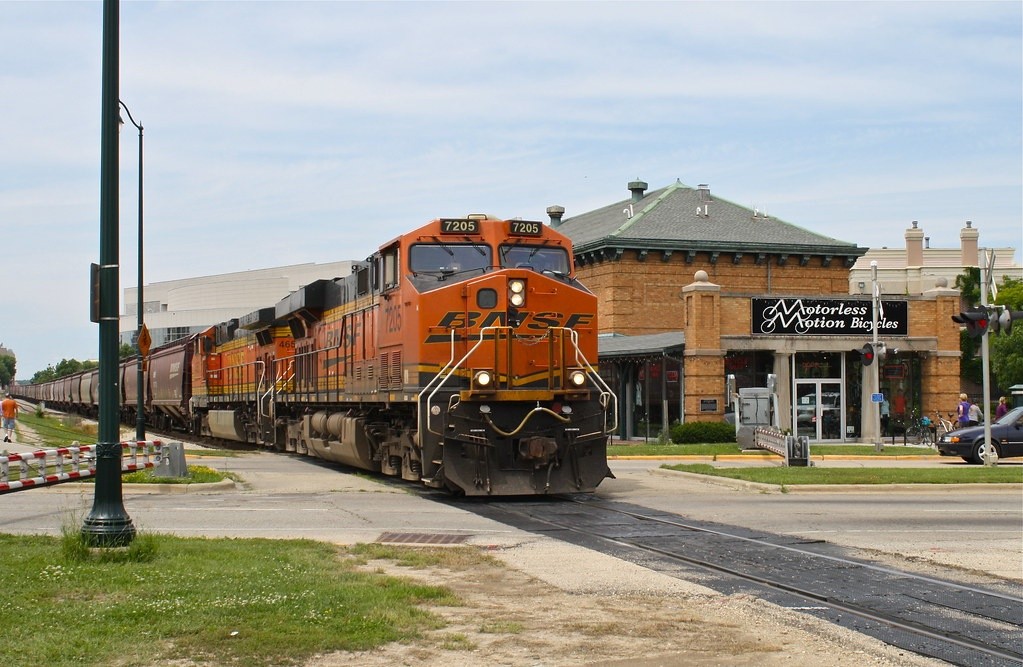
[8,213,617,502]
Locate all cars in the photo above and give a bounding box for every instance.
[936,404,1022,465]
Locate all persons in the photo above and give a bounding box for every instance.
[892,389,906,420]
[956,394,971,428]
[968,398,983,426]
[2,393,18,443]
[880,391,890,437]
[0,402,3,428]
[996,397,1008,420]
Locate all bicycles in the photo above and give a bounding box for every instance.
[907,409,959,448]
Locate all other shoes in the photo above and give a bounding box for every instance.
[3,434,8,442]
[8,438,11,442]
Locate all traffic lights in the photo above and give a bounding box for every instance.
[851,344,874,364]
[950,312,987,337]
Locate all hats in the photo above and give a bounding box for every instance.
[971,398,979,403]
